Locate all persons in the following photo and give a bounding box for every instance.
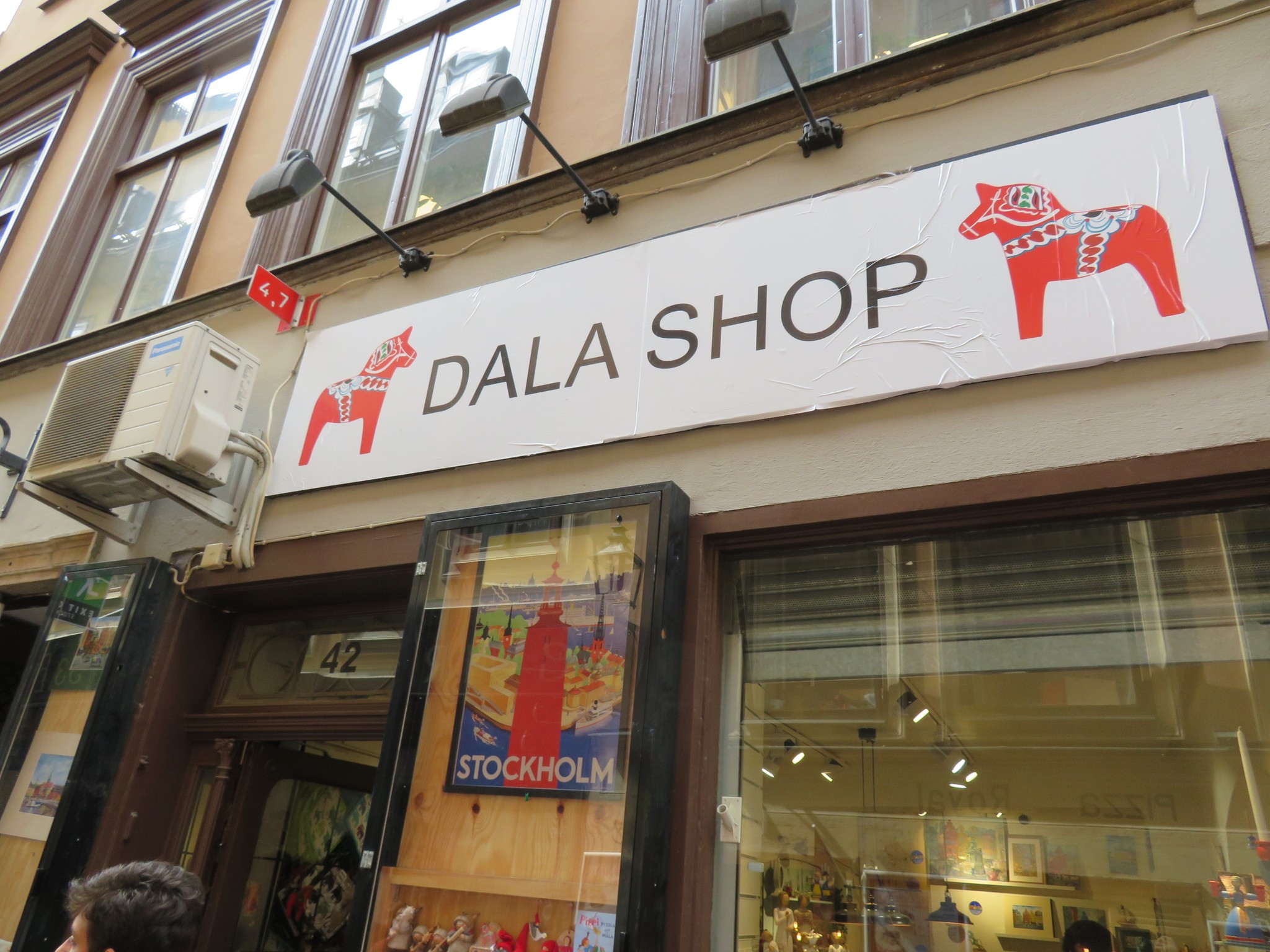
[1219,875,1267,946]
[53,860,210,952]
[1061,919,1112,952]
[828,932,844,952]
[773,891,797,952]
[762,930,779,952]
[794,894,813,932]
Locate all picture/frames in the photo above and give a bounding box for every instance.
[1006,834,1047,885]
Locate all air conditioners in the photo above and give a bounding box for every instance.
[20,320,262,509]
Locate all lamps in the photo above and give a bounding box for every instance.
[762,738,805,778]
[934,742,977,790]
[702,0,844,157]
[895,692,930,723]
[831,888,913,927]
[820,759,843,783]
[246,147,435,277]
[923,736,975,926]
[439,73,619,223]
